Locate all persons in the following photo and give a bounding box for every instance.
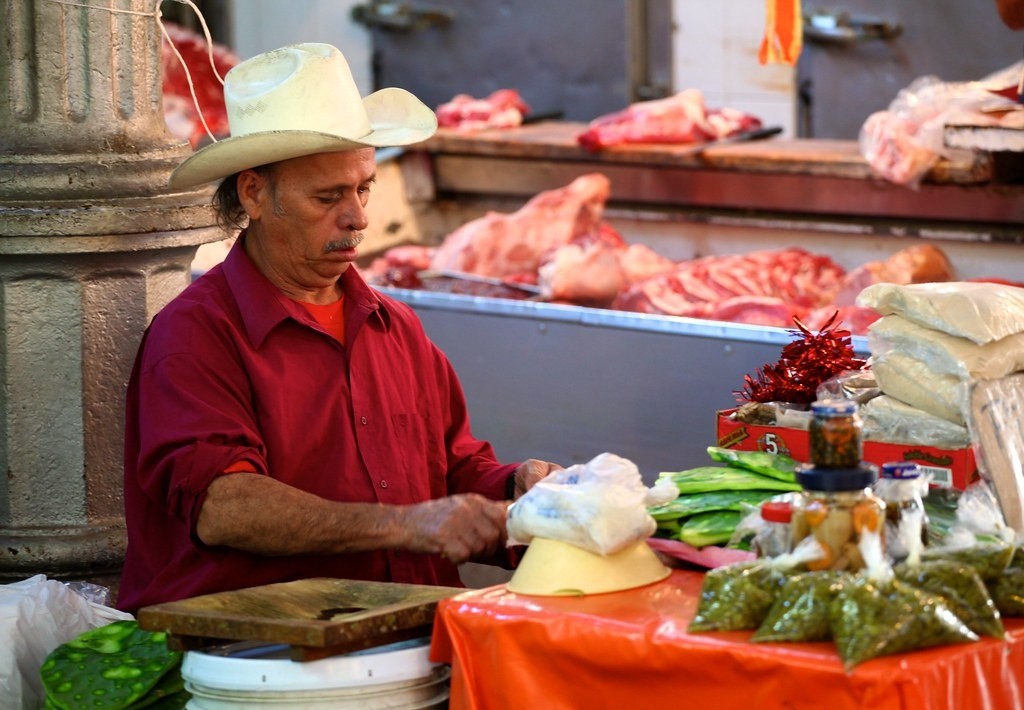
[115,42,568,616]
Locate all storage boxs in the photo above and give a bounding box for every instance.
[715,403,993,489]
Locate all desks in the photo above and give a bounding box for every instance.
[428,568,1024,710]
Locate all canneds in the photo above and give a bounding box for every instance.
[789,399,886,576]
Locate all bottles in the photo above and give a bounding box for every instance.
[758,501,794,554]
[877,462,925,558]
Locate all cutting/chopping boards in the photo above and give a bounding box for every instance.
[406,121,1002,183]
[139,578,477,645]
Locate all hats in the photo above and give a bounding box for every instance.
[169,42,438,190]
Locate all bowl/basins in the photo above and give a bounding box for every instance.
[506,536,672,596]
